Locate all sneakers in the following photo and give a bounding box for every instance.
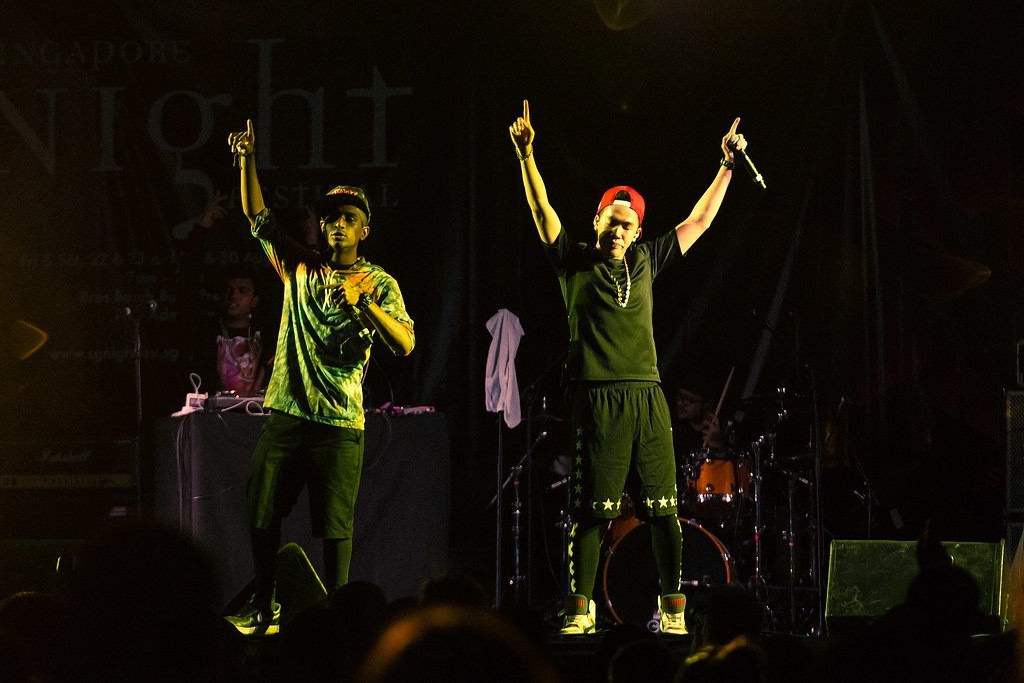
[224,602,280,635]
[556,593,596,635]
[657,593,687,636]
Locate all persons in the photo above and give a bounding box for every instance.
[186,190,319,392]
[0,521,1024,683]
[510,100,747,638]
[667,371,729,452]
[227,120,415,635]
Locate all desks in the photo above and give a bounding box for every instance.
[156,413,446,601]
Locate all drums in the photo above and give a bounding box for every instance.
[601,510,733,625]
[683,448,754,520]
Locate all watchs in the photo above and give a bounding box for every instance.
[356,292,372,310]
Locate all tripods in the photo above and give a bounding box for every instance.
[748,396,819,635]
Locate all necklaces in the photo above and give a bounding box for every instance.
[221,321,254,378]
[608,256,631,308]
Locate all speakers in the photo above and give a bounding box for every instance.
[0,438,143,552]
[216,542,329,627]
[824,537,1010,634]
[1002,387,1024,559]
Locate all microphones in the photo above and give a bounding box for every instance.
[342,305,374,346]
[727,140,767,191]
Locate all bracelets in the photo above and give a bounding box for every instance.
[720,158,734,169]
[233,148,256,167]
[515,149,534,161]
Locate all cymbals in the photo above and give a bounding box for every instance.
[742,392,808,409]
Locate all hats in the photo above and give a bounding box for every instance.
[597,186,646,229]
[324,186,371,224]
